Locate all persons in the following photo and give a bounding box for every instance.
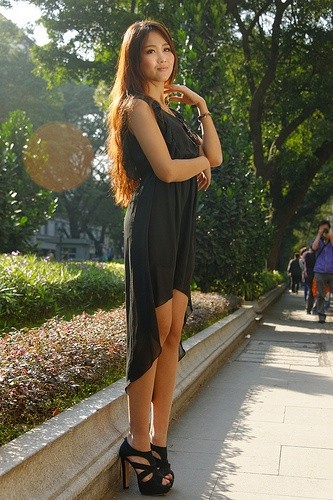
[105,20,222,496]
[287,221,333,323]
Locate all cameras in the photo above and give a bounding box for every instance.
[324,229,329,233]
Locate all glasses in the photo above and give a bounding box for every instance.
[179,118,201,147]
[323,228,329,234]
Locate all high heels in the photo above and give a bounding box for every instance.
[119,436,171,496]
[150,442,174,488]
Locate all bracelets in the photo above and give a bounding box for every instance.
[197,113,212,122]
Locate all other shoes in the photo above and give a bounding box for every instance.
[319,315,325,322]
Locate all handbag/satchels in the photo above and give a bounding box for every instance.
[307,266,314,289]
